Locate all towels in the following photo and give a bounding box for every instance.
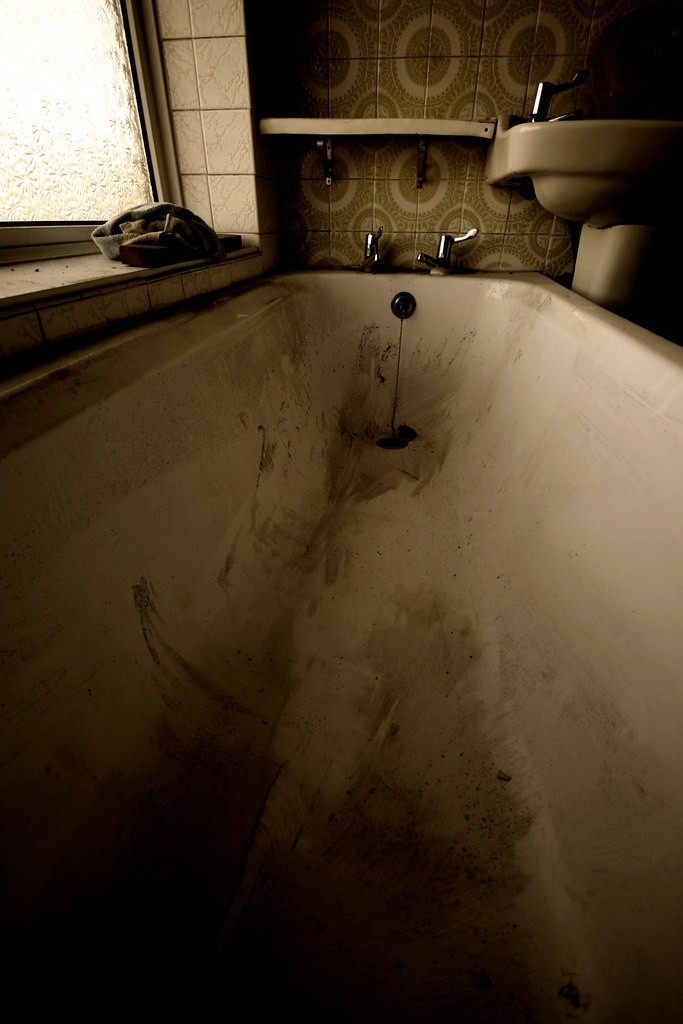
[91,202,228,264]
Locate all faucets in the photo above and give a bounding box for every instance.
[363,226,383,272]
[416,228,480,277]
[529,71,586,122]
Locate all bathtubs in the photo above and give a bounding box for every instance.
[0,275,682,1024]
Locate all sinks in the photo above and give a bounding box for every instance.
[486,120,683,224]
